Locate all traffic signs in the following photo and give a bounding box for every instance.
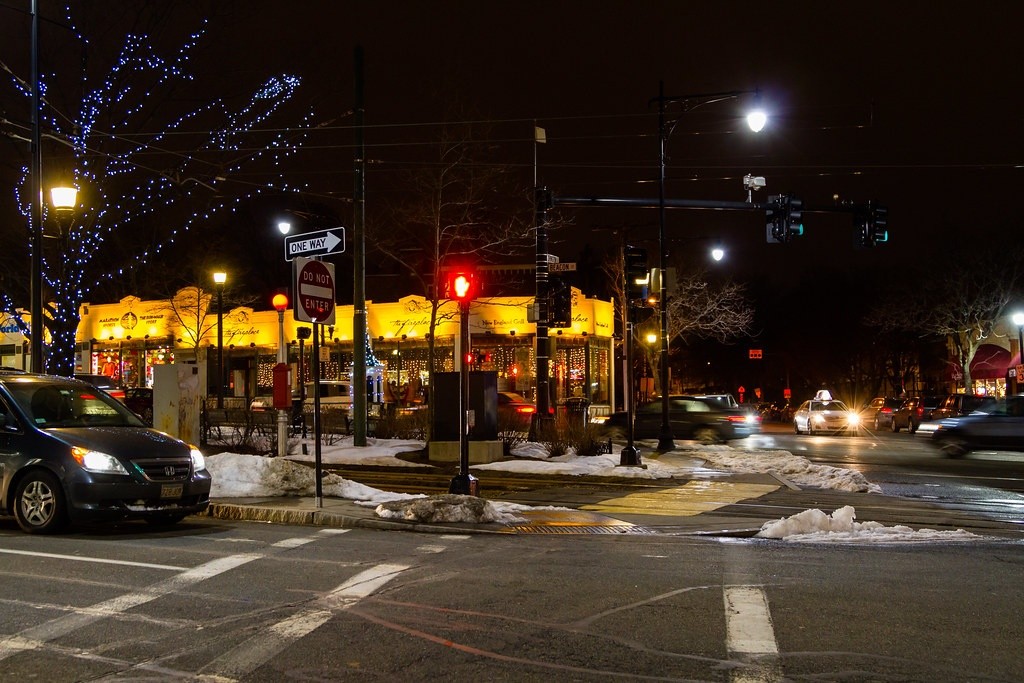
[284,227,345,261]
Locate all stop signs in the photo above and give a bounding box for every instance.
[299,261,335,323]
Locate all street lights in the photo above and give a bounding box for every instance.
[31,173,79,376]
[1014,313,1024,393]
[657,90,768,453]
[212,270,227,410]
[648,331,657,393]
[273,294,288,456]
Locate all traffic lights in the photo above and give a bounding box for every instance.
[781,197,804,235]
[870,208,887,245]
[449,272,476,300]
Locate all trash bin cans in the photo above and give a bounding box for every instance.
[564,397,591,426]
[125,387,153,428]
[380,402,395,418]
[288,400,302,425]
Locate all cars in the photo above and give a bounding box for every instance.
[859,397,903,431]
[793,399,858,437]
[705,393,739,409]
[0,375,212,535]
[916,395,1024,458]
[69,373,126,427]
[122,388,153,425]
[891,396,942,434]
[496,391,536,432]
[923,393,997,422]
[605,395,762,445]
